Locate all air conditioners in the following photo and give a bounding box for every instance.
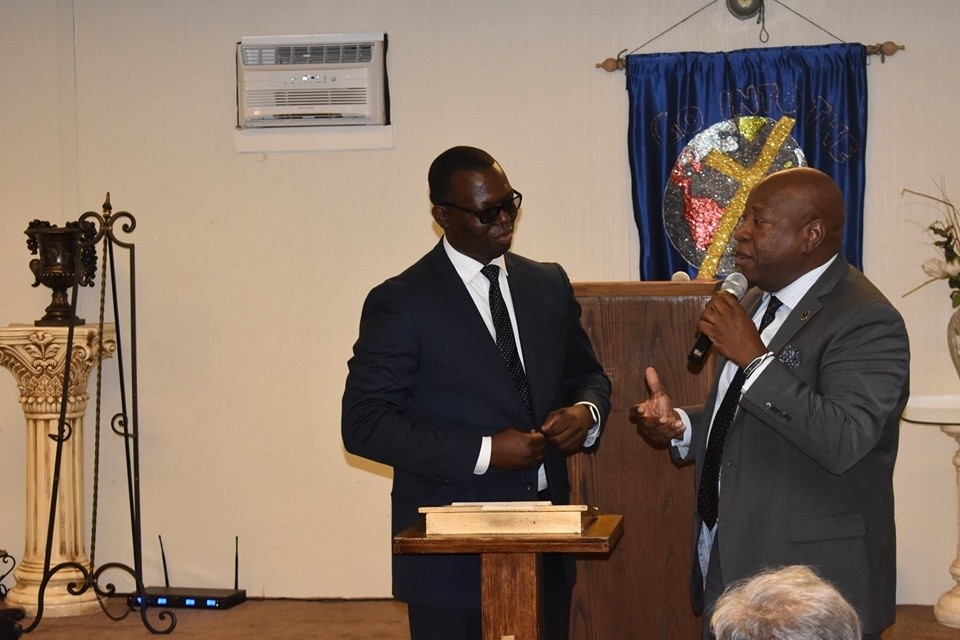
[233,32,386,127]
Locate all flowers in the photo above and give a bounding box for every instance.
[900,185,960,299]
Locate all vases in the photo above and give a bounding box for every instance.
[947,290,960,374]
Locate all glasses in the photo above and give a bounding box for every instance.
[440,190,523,224]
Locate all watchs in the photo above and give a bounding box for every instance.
[582,404,599,430]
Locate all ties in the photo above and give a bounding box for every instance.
[698,295,782,532]
[480,264,543,472]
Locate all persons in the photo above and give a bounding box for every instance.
[709,563,863,640]
[633,166,911,640]
[341,144,613,640]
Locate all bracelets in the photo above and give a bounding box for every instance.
[743,350,775,380]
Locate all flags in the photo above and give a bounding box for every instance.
[625,41,868,283]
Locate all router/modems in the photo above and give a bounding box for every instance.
[126,535,247,610]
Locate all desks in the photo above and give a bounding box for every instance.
[392,512,623,640]
[900,407,960,628]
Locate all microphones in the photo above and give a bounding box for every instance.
[672,271,691,282]
[687,272,749,365]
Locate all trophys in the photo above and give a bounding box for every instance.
[24,217,99,325]
